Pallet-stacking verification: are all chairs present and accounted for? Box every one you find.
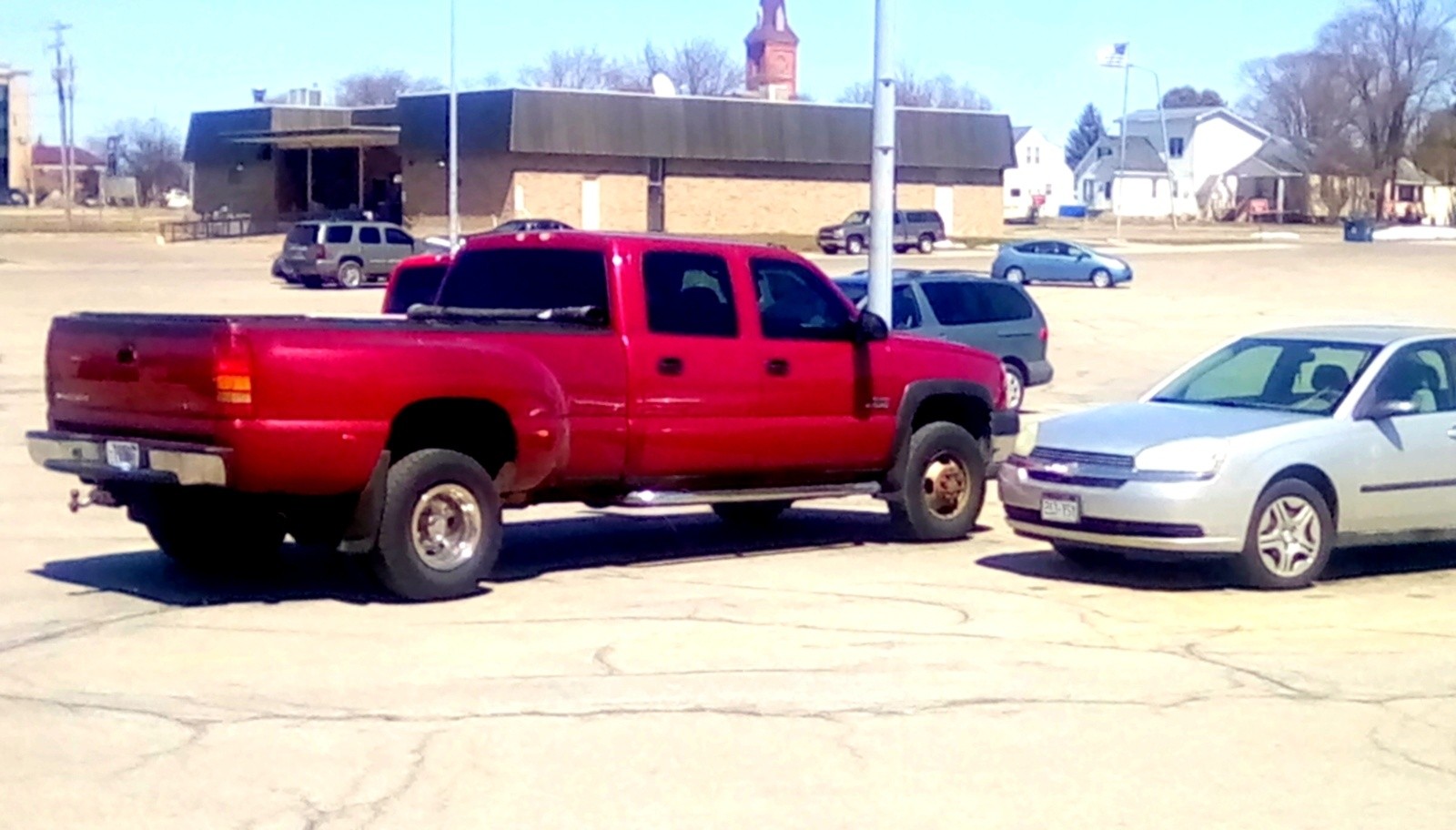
[1311,364,1349,390]
[681,287,719,305]
[1403,362,1440,391]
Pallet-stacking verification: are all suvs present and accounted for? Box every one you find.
[273,219,575,289]
[817,210,947,254]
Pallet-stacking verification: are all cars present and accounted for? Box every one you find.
[991,240,1133,288]
[997,326,1456,589]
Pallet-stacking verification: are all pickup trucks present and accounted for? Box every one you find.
[25,232,1020,603]
